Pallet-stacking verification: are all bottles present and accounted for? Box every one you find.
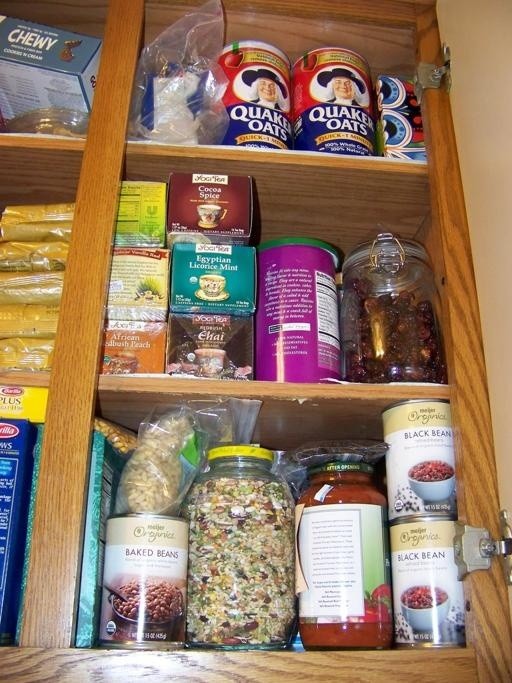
[340,231,447,385]
[188,444,295,651]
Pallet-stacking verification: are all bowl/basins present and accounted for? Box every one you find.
[398,587,449,631]
[408,461,457,502]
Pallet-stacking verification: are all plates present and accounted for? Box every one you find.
[8,106,89,140]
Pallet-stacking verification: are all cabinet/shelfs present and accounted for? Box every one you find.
[0,0,512,683]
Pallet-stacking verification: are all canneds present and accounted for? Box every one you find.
[214,39,290,149]
[98,514,190,651]
[290,47,379,157]
